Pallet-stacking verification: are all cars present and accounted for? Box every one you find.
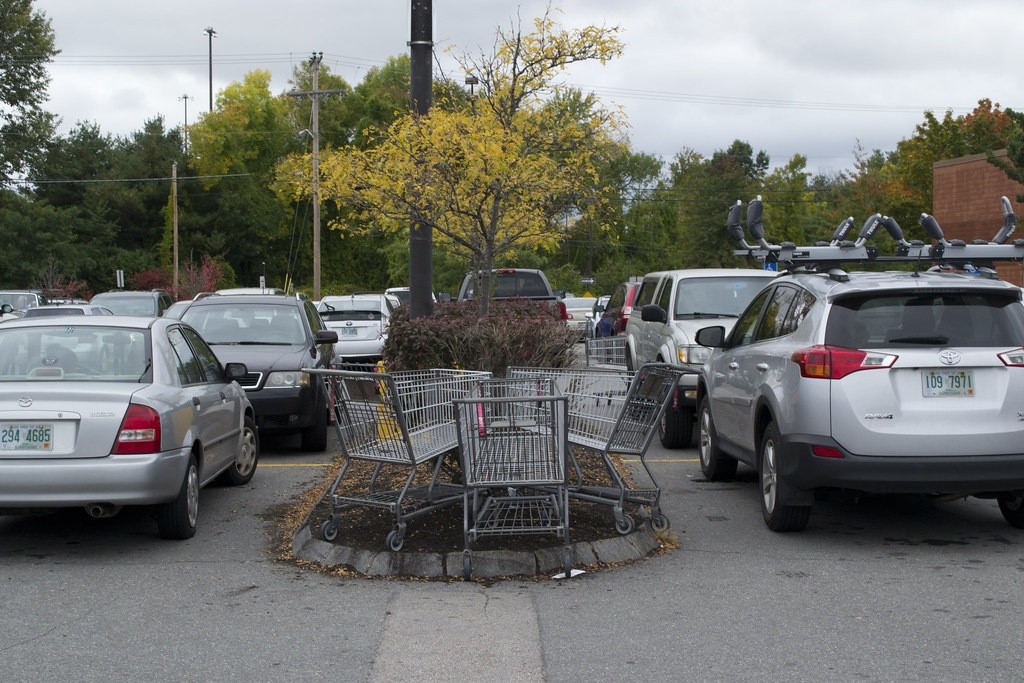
[385,294,403,308]
[0,316,259,540]
[561,298,597,337]
[22,305,115,316]
[162,300,193,319]
[385,286,437,304]
[593,281,644,366]
[317,294,394,370]
[585,295,612,341]
[177,291,337,454]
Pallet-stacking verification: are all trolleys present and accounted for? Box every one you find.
[301,356,491,553]
[450,372,574,581]
[505,365,705,535]
[585,335,625,368]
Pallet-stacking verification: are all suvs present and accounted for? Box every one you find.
[625,268,792,449]
[0,289,48,312]
[697,196,1024,533]
[88,289,173,317]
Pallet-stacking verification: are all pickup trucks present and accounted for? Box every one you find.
[438,267,567,327]
